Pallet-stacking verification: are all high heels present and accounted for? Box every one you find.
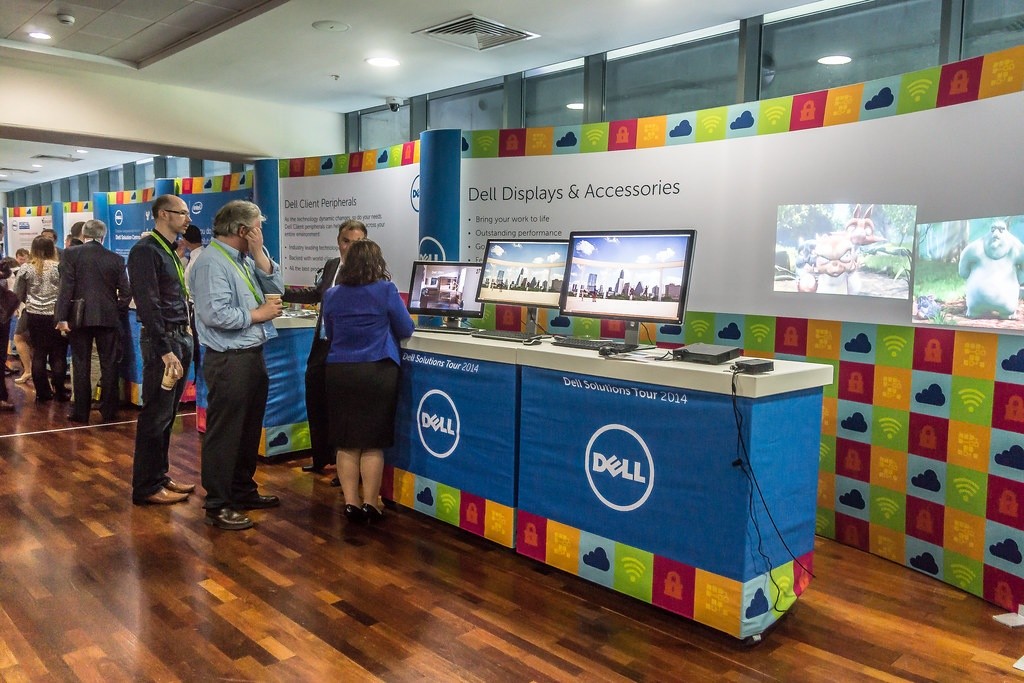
[359,503,388,530]
[344,503,361,531]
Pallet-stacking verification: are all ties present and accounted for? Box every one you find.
[320,266,343,340]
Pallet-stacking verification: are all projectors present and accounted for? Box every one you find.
[672,342,740,364]
[736,359,774,373]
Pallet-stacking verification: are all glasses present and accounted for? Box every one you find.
[165,210,191,217]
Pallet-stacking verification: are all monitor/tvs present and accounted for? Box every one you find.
[475,239,569,334]
[406,261,485,327]
[560,229,697,350]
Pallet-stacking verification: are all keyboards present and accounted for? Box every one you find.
[472,330,542,342]
[550,338,639,353]
[414,326,479,334]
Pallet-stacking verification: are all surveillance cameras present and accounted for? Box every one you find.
[385,97,403,112]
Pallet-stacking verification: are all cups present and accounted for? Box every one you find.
[161,368,179,391]
[264,294,281,302]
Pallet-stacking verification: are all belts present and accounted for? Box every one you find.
[142,320,186,333]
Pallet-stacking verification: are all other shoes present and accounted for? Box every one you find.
[0,400,16,412]
[14,371,32,383]
[100,407,117,423]
[36,385,72,402]
[66,412,89,425]
[4,364,19,376]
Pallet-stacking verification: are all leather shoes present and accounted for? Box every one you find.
[132,486,190,505]
[230,492,279,509]
[302,464,323,472]
[330,476,341,487]
[203,507,254,530]
[164,480,196,493]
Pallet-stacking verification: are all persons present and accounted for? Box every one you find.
[320,240,414,523]
[14,234,70,401]
[41,222,100,402]
[55,220,132,424]
[281,221,368,487]
[127,194,196,504]
[0,222,31,410]
[189,201,285,530]
[177,225,206,384]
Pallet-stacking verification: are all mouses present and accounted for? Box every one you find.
[599,347,612,355]
[523,339,542,345]
